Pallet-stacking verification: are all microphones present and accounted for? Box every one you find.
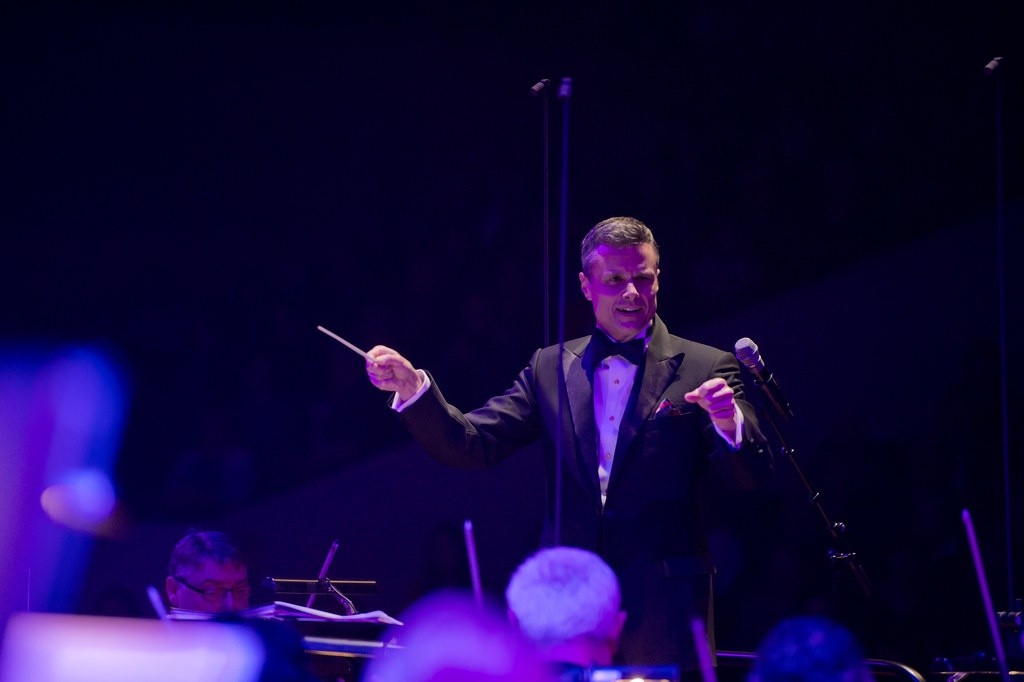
[735,337,793,420]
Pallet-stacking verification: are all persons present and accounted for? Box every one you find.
[366,214,776,680]
[505,545,630,668]
[163,532,249,615]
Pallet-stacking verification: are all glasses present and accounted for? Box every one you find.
[174,576,250,604]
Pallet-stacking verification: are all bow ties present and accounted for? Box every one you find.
[586,330,645,367]
